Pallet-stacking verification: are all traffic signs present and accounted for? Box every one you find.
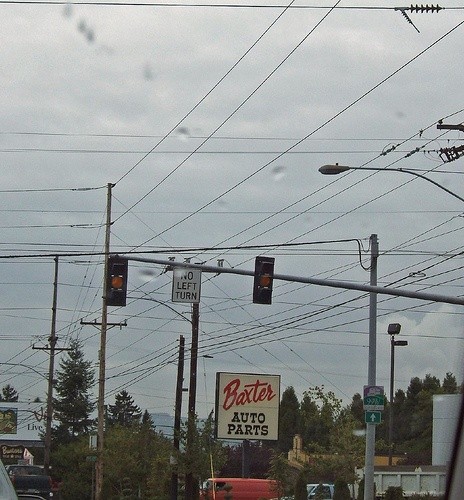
[171,268,201,303]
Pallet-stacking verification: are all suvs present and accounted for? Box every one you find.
[280,482,350,500]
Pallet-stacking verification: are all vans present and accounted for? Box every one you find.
[198,478,283,500]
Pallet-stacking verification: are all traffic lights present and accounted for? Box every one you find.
[252,256,275,305]
[105,257,128,307]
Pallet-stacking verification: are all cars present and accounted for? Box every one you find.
[3,465,52,499]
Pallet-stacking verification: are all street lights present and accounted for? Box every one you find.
[387,323,408,466]
[316,163,464,205]
